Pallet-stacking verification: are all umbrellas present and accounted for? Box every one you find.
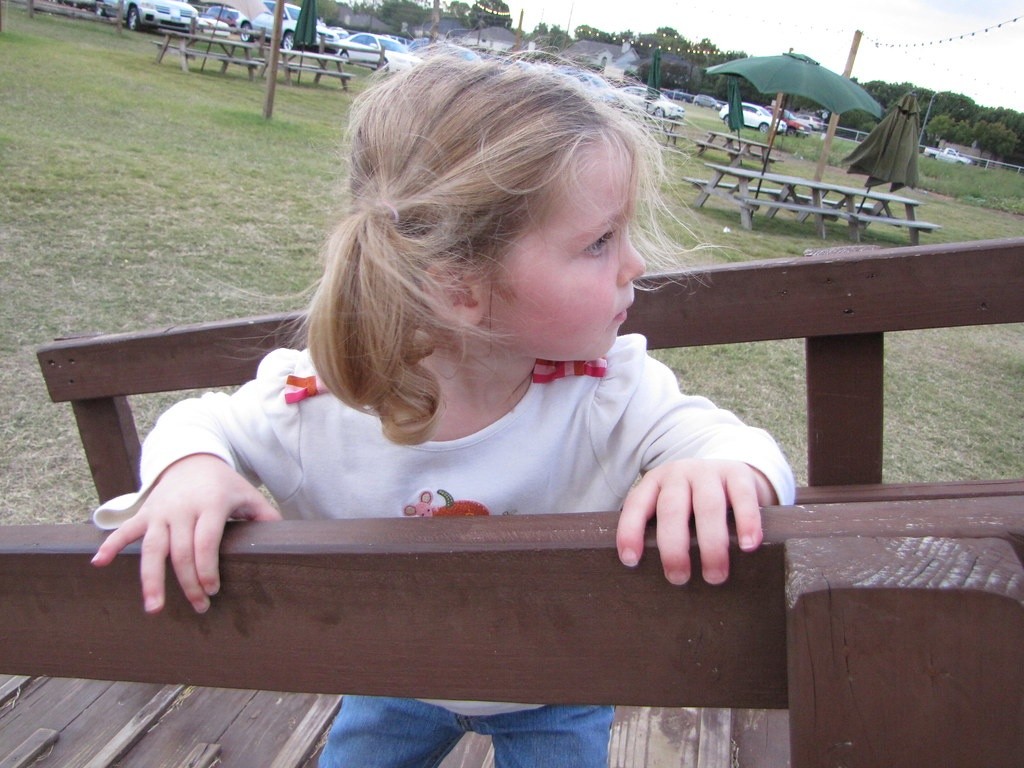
[294,0,317,83]
[643,48,661,118]
[195,0,276,73]
[726,76,745,167]
[838,89,922,214]
[703,53,882,220]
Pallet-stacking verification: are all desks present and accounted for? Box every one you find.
[156,27,255,82]
[693,162,838,240]
[259,45,349,91]
[699,130,774,174]
[797,182,926,245]
[645,109,686,146]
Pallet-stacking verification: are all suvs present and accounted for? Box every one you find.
[235,0,341,55]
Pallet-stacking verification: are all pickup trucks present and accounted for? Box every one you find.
[924,146,971,166]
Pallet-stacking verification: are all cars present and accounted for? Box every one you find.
[693,94,722,111]
[796,114,824,132]
[330,23,479,72]
[504,48,694,121]
[718,102,788,135]
[764,105,811,139]
[197,6,239,38]
[96,0,200,33]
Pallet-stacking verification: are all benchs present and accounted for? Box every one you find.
[152,40,942,232]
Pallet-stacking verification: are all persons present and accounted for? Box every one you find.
[89,37,797,767]
